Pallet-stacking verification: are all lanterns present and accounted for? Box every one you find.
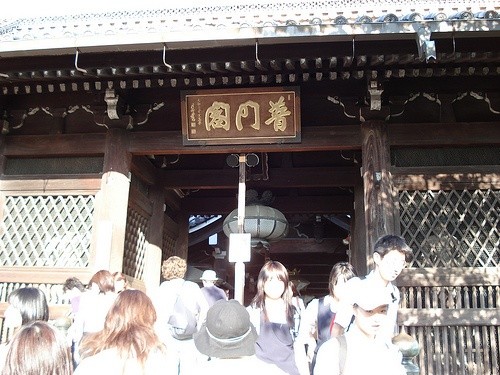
[223,205,288,251]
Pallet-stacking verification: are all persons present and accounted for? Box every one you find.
[313,280,407,375]
[0,256,358,375]
[330,234,413,346]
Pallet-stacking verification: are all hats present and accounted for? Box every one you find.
[199,270,218,281]
[351,280,392,312]
[193,299,257,357]
